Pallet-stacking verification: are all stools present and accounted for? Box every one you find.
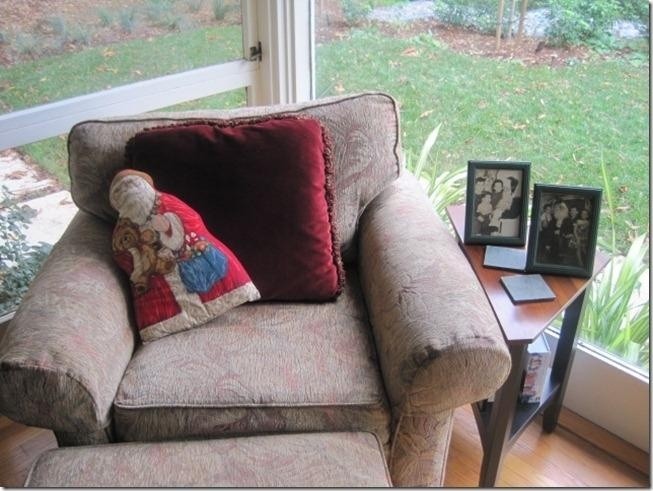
[23,430,393,490]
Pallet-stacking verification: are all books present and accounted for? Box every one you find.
[515,333,551,406]
[499,273,556,305]
[482,246,527,274]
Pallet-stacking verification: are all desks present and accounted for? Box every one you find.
[446,205,611,487]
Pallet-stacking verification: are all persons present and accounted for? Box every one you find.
[472,176,522,237]
[537,201,591,265]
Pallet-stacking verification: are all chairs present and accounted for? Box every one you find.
[1,92,514,486]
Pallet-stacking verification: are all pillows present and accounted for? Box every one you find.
[121,116,344,303]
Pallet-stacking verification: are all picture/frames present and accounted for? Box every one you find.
[465,160,604,279]
